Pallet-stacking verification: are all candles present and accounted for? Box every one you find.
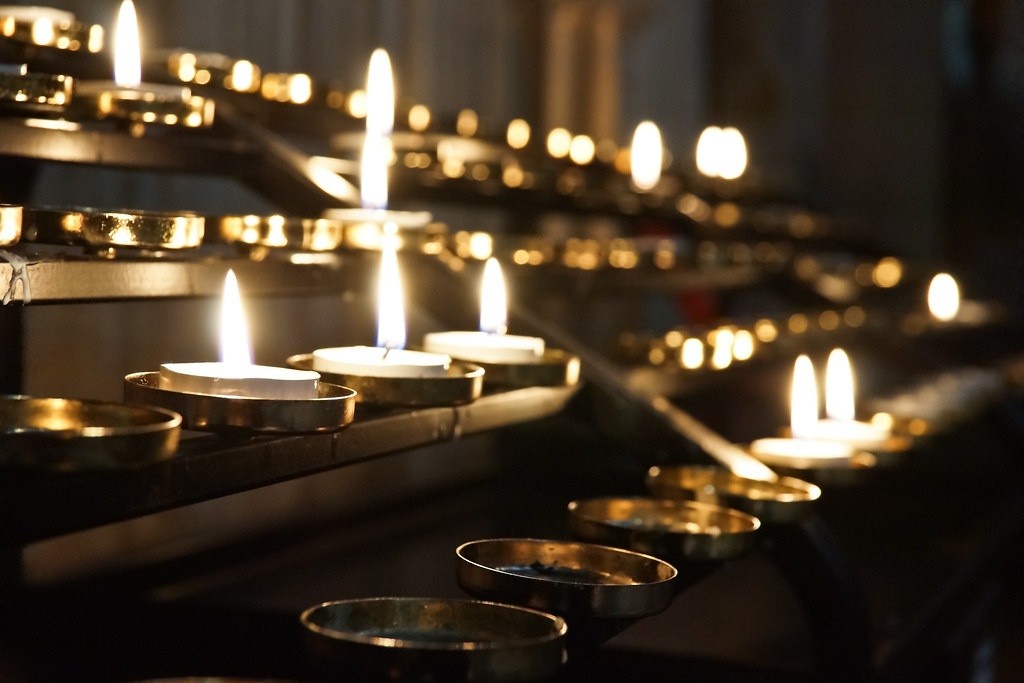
[749,339,882,467]
[325,131,429,250]
[424,257,546,364]
[161,268,322,400]
[76,0,191,103]
[311,250,454,379]
